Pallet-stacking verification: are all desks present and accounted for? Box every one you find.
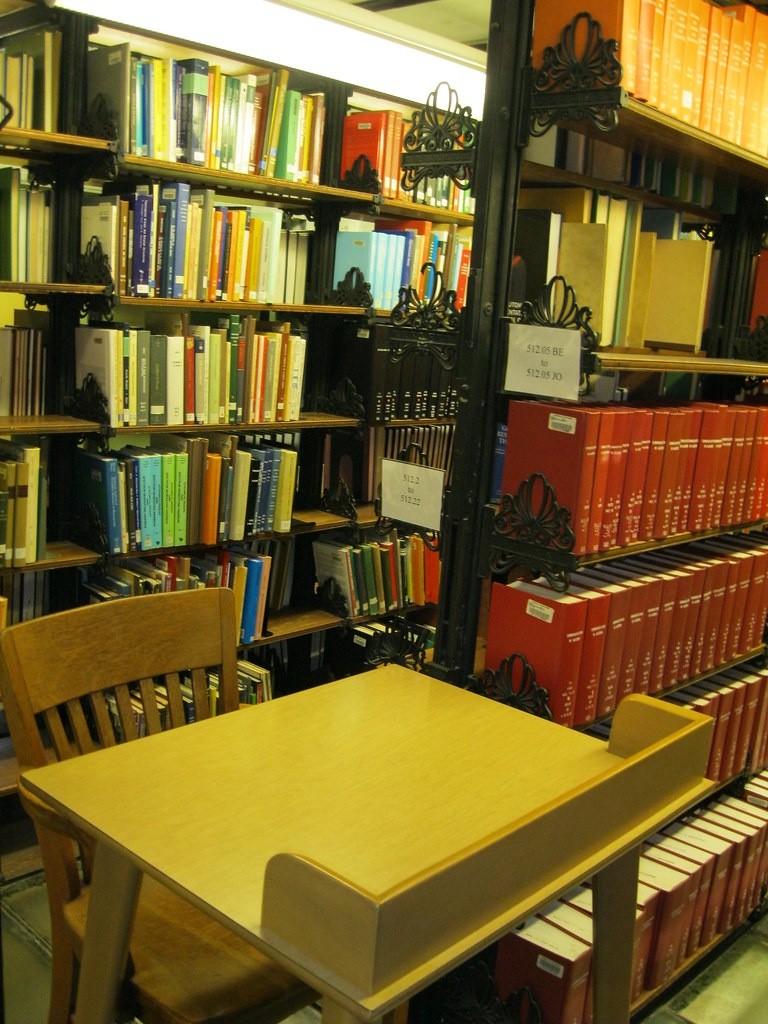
[18,664,719,1024]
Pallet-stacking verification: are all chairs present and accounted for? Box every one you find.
[0,588,413,1024]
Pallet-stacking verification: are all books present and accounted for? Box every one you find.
[1,0,768,1024]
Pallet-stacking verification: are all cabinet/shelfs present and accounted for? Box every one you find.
[0,1,489,887]
[421,1,767,1024]
